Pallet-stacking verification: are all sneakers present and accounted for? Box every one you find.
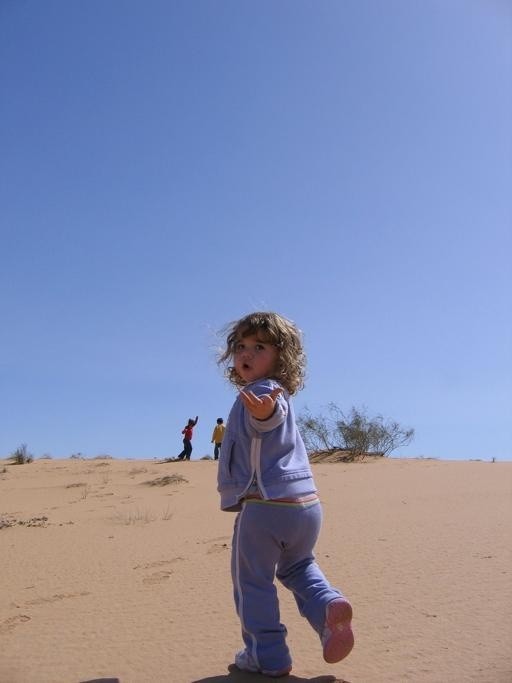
[235,650,292,676]
[320,598,354,663]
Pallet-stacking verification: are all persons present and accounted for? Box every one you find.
[175,415,201,460]
[210,416,226,460]
[213,309,356,677]
